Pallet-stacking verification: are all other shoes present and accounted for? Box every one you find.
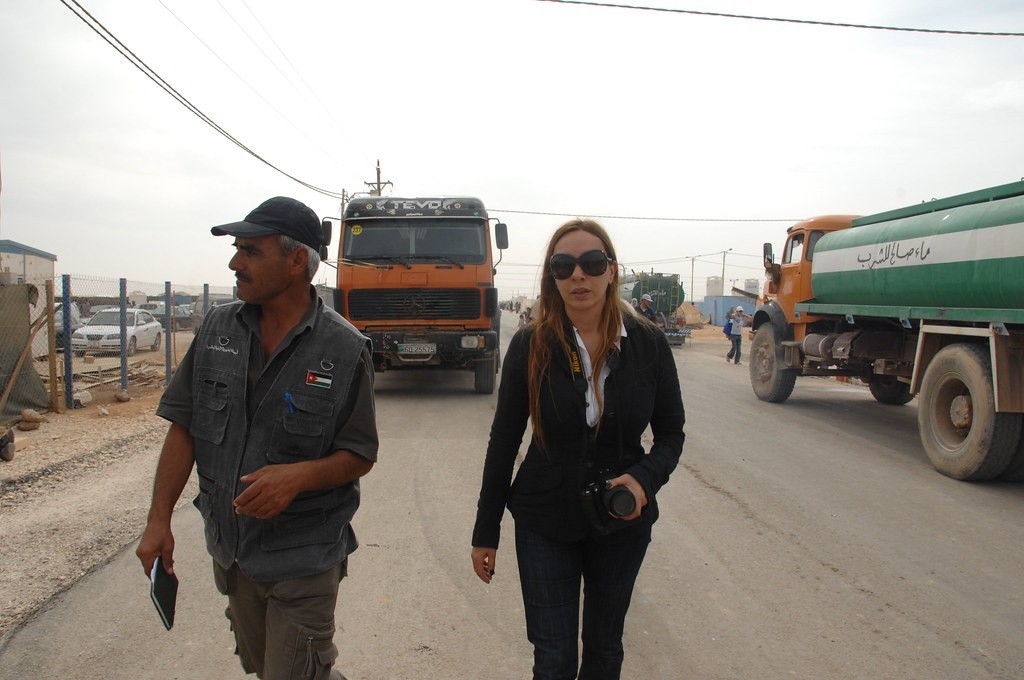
[736,362,742,365]
[726,355,730,362]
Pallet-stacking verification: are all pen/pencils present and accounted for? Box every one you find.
[284,392,294,414]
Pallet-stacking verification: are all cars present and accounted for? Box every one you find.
[53,311,86,348]
[71,307,162,357]
[151,306,193,332]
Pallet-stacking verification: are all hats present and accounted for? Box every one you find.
[211,196,321,252]
[642,294,653,302]
[737,306,743,312]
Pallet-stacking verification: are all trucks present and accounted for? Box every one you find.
[318,196,509,395]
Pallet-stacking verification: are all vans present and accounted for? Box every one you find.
[133,304,157,312]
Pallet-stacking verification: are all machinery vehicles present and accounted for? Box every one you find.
[748,178,1023,483]
[618,272,692,346]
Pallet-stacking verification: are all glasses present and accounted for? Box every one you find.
[549,250,613,280]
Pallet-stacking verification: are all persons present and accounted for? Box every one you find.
[471,218,686,680]
[635,294,656,324]
[501,301,521,314]
[135,197,379,679]
[518,307,532,328]
[726,306,744,365]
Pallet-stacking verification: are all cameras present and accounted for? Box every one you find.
[583,469,636,517]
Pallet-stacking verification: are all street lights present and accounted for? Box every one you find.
[686,254,701,302]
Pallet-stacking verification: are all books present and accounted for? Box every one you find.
[149,558,179,631]
